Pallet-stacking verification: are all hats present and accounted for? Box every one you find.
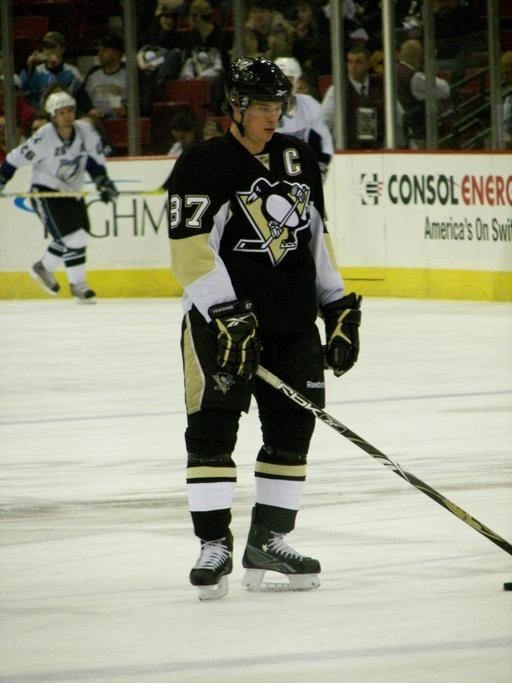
[92,33,124,54]
[40,31,65,45]
[169,112,193,129]
[180,0,212,15]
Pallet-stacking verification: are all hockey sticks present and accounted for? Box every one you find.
[1,173,172,199]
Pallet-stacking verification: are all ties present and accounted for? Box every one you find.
[360,85,366,95]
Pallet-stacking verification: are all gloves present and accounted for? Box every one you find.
[208,299,260,393]
[318,291,362,376]
[97,179,118,203]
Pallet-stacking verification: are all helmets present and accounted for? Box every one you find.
[275,57,301,78]
[155,1,181,17]
[46,92,75,115]
[224,57,293,121]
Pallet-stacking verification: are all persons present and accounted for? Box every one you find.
[275,56,334,191]
[0,0,511,152]
[156,55,363,586]
[2,89,121,304]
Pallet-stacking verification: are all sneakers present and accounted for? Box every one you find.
[70,281,95,299]
[242,528,320,574]
[32,262,60,292]
[189,531,233,586]
